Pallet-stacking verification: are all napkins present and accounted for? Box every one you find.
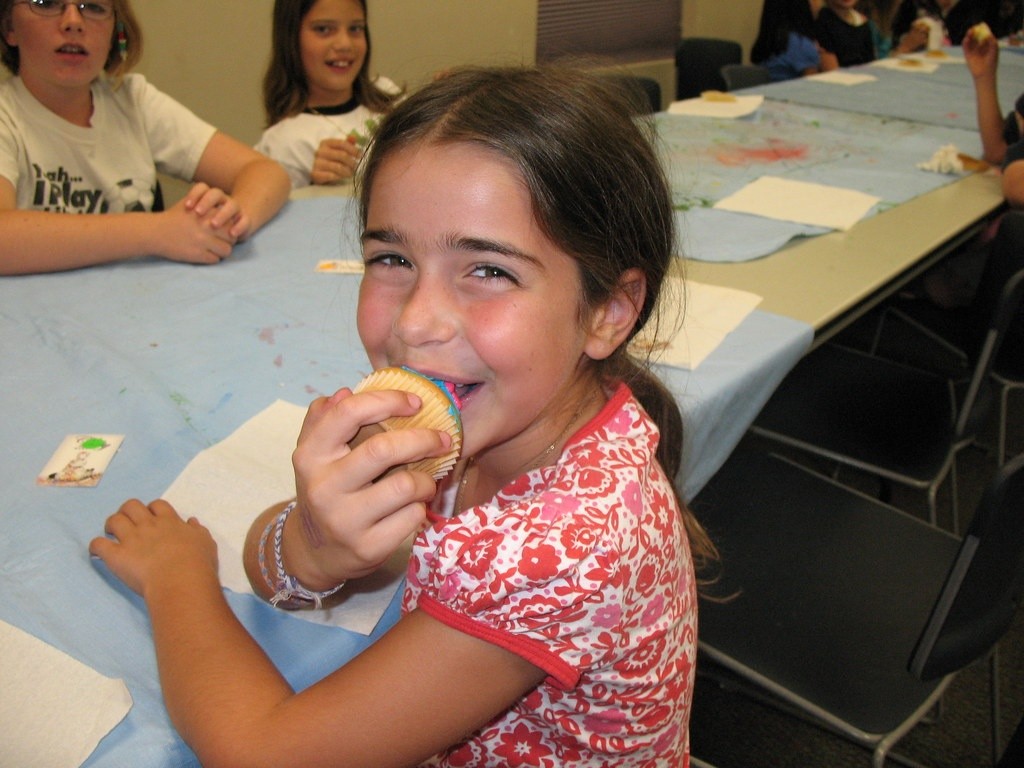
[668,94,764,118]
[618,273,763,372]
[803,68,878,86]
[914,17,943,49]
[712,173,885,233]
[904,52,966,65]
[0,616,136,768]
[160,398,415,636]
[875,57,939,74]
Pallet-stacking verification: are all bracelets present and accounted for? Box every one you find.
[258,502,345,608]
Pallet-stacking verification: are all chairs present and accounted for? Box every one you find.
[684,448,1024,768]
[867,208,1024,470]
[714,215,1024,768]
[750,266,1024,533]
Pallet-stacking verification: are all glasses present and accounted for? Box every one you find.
[13,0,114,20]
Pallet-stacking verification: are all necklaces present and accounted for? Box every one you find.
[310,105,349,137]
[457,383,597,514]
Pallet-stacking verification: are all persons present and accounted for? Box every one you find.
[83,63,721,768]
[749,0,1024,304]
[250,0,413,190]
[0,0,291,276]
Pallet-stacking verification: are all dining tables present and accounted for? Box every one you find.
[0,42,1024,768]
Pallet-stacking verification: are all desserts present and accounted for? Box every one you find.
[345,366,463,481]
